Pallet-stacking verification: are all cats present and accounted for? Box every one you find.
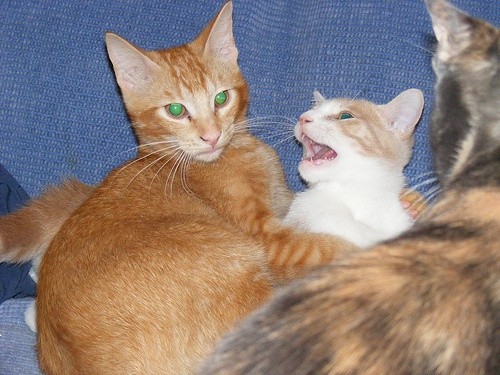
[33,0,360,375]
[0,87,427,338]
[197,1,500,375]
